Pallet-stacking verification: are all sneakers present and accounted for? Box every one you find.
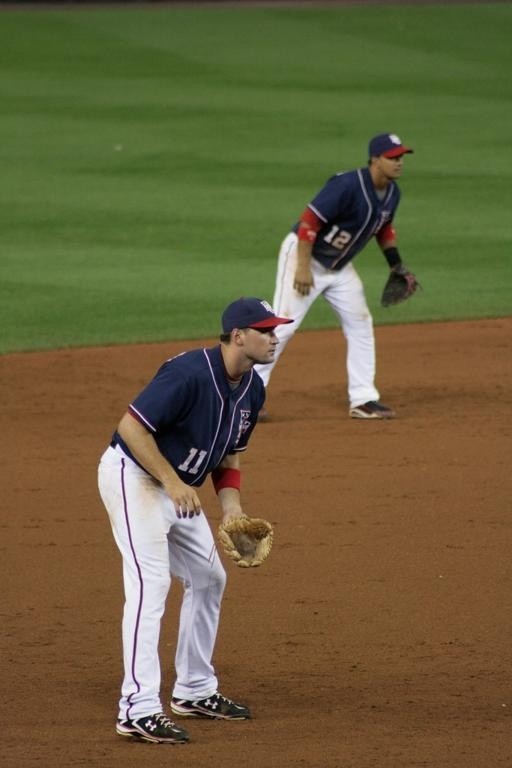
[114,711,189,746]
[349,400,395,420]
[171,691,250,722]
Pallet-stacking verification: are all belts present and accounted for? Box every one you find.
[109,436,117,448]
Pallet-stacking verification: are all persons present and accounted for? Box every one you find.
[96,293,296,744]
[248,130,418,419]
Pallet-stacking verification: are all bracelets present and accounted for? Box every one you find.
[380,244,401,266]
[210,466,244,495]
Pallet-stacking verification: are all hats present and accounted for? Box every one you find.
[222,297,295,333]
[368,132,414,159]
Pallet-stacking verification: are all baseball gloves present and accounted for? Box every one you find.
[382,267,416,307]
[218,514,273,568]
